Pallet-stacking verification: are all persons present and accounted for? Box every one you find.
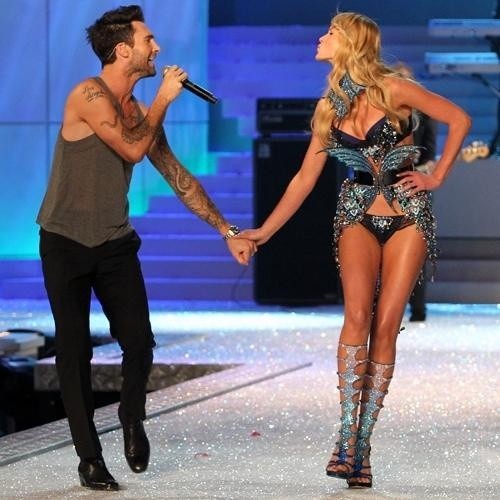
[234,12,473,491]
[38,5,258,491]
[395,58,437,322]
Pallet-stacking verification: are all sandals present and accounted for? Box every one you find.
[325,442,372,489]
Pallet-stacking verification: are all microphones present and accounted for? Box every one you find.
[163,66,218,105]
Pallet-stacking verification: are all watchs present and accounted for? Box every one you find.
[222,225,240,242]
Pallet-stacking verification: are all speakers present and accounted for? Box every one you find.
[253,133,345,305]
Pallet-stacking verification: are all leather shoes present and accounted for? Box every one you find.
[78,458,118,491]
[122,421,150,474]
